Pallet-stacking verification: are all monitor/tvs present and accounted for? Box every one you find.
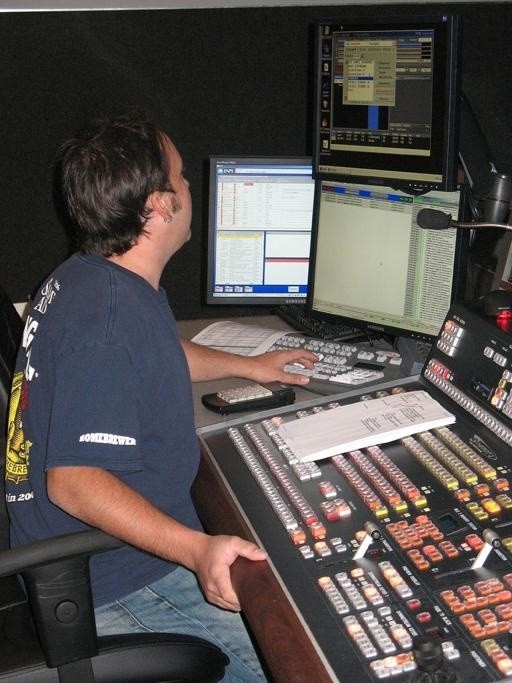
[312,14,464,193]
[305,189,466,345]
[207,155,315,305]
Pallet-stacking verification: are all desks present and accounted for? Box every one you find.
[176,315,512,683]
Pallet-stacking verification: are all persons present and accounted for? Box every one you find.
[4,114,319,682]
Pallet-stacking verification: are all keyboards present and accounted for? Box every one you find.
[267,333,403,397]
[275,305,378,341]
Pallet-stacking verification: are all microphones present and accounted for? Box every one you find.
[417,209,512,230]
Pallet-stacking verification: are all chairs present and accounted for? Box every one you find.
[0,284,231,683]
